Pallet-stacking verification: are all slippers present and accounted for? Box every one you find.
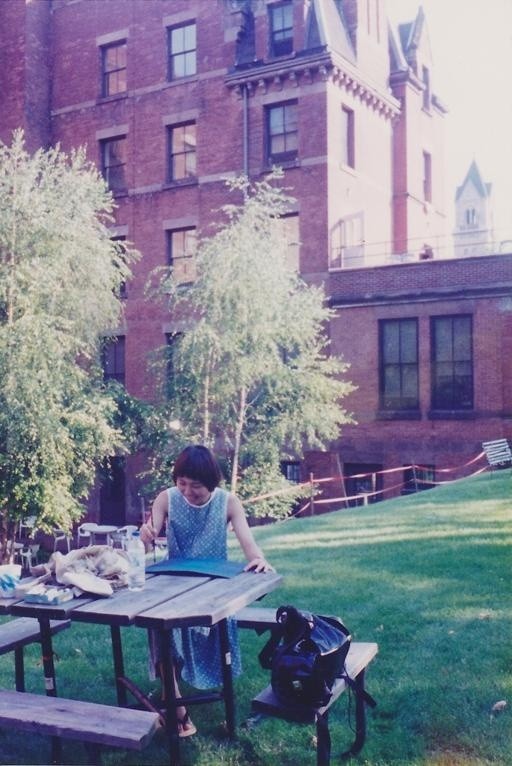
[160,703,198,740]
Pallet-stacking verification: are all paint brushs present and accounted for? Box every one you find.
[150,507,157,566]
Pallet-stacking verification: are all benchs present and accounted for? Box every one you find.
[235,607,287,629]
[0,690,161,766]
[0,616,71,692]
[250,641,379,766]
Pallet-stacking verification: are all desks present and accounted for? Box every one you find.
[0,560,284,766]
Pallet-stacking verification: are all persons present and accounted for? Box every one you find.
[141,445,277,738]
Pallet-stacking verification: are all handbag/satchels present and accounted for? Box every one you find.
[256,602,377,723]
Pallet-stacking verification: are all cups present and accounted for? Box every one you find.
[0,564,22,598]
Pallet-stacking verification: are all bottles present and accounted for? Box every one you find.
[127,532,147,591]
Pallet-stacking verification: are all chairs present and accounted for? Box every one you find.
[6,517,139,571]
